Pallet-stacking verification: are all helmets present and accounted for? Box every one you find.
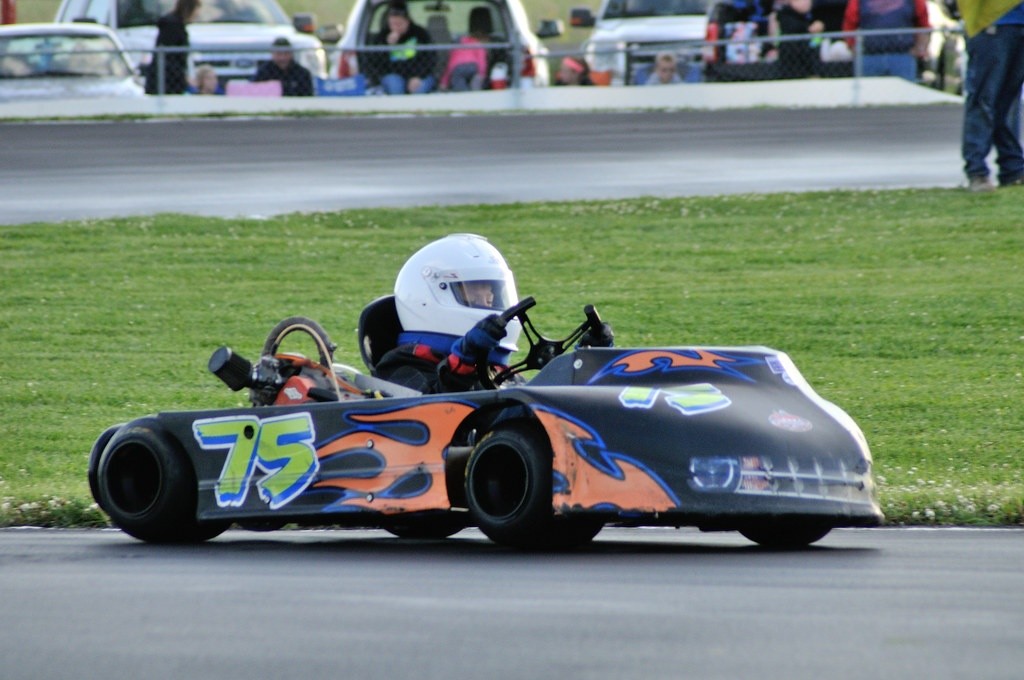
[394,234,522,365]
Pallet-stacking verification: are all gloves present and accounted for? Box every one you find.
[574,322,614,352]
[451,314,507,361]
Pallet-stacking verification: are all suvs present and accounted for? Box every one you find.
[53,0,344,82]
[568,0,721,87]
[318,0,565,95]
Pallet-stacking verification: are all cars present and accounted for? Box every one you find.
[0,22,147,101]
[704,0,969,94]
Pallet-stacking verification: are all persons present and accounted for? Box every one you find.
[197,65,217,94]
[252,39,314,97]
[145,0,202,94]
[703,0,931,84]
[932,0,1024,190]
[366,233,614,399]
[368,6,439,94]
[647,50,682,85]
[440,33,490,91]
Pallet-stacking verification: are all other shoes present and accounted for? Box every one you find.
[971,178,994,191]
[1007,177,1024,186]
